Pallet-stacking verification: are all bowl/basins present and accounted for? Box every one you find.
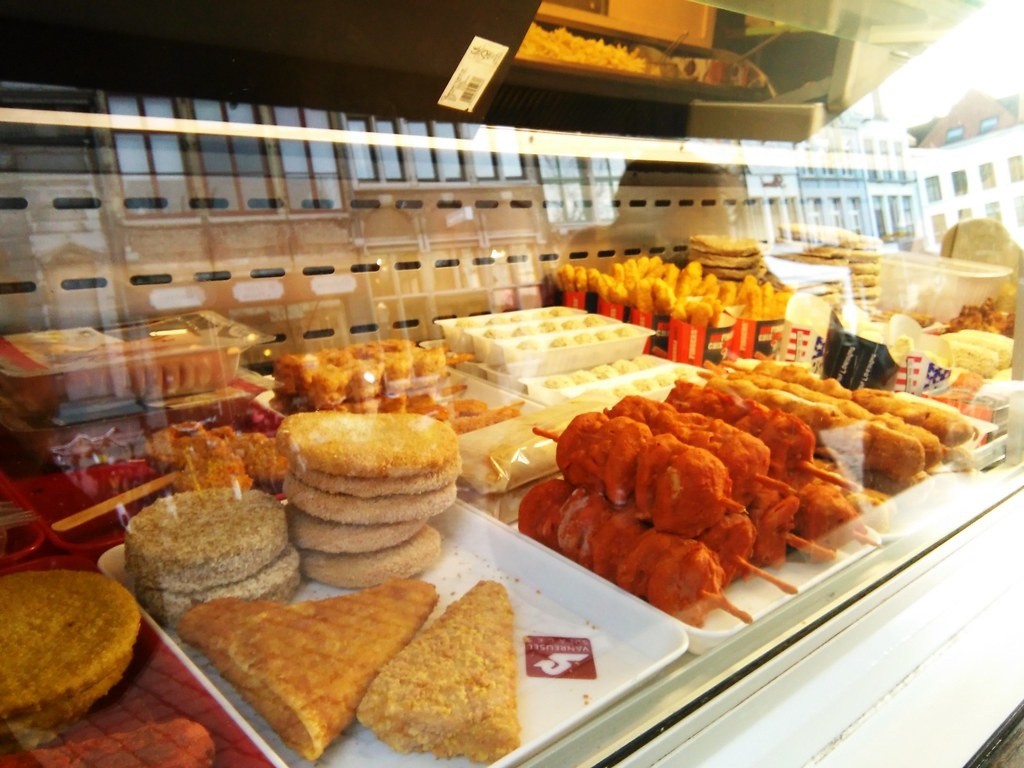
[878,252,1013,324]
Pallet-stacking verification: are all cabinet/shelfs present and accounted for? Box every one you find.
[4,107,1024,768]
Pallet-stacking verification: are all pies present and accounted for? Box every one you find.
[0,411,518,768]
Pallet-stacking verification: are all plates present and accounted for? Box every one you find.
[0,482,45,564]
[99,498,688,768]
[510,519,882,655]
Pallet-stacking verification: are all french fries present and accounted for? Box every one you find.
[552,256,786,332]
